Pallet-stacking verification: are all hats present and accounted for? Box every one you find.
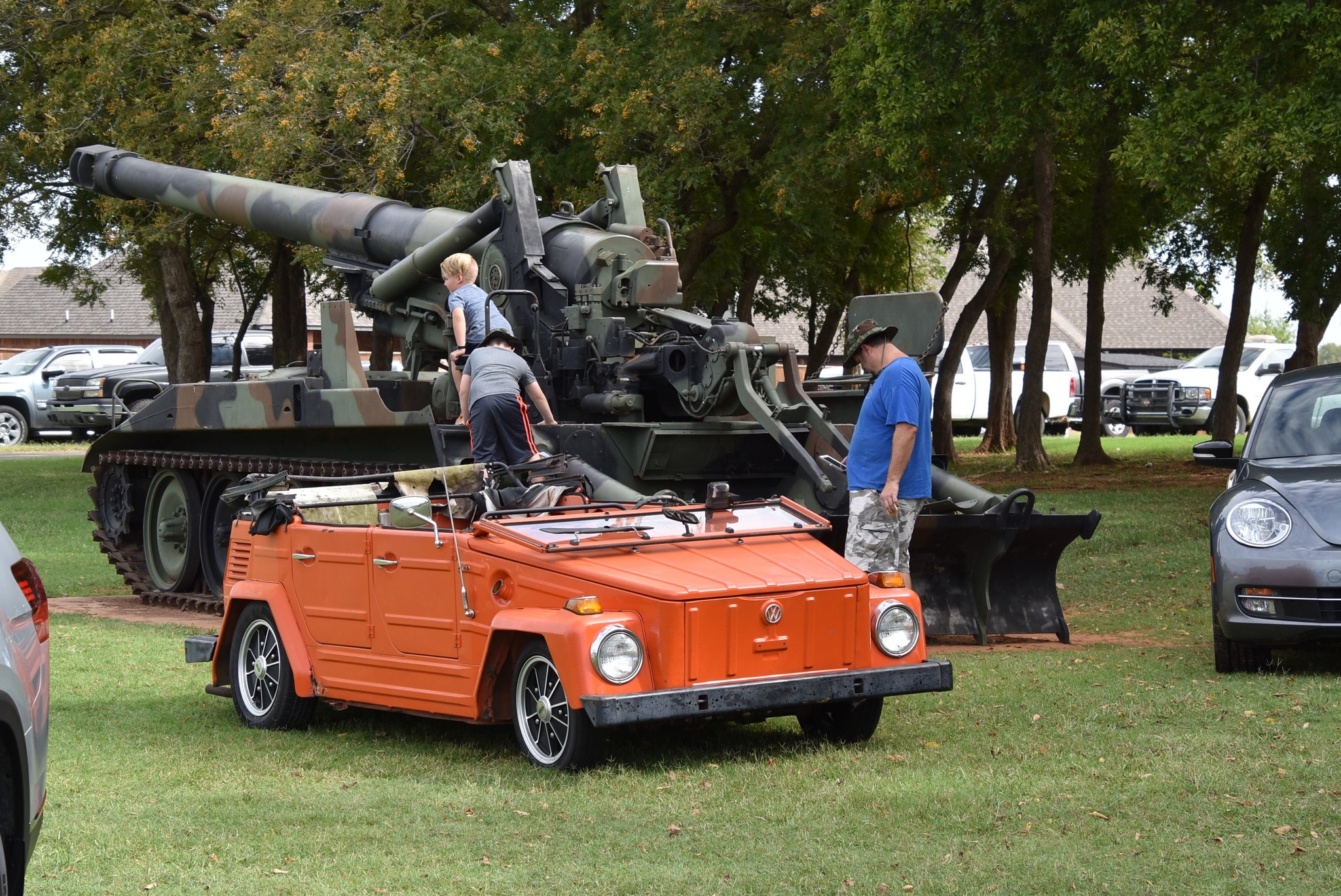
[482,328,524,356]
[842,318,898,371]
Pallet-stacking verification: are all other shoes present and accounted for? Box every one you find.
[455,413,466,426]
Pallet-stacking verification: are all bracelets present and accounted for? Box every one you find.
[456,345,466,349]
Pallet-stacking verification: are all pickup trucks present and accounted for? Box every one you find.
[820,342,1150,437]
[1122,336,1341,436]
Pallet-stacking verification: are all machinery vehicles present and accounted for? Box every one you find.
[62,136,1101,645]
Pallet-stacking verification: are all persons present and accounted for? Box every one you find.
[440,253,515,425]
[833,319,933,593]
[459,332,559,488]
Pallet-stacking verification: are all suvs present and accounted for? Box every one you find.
[0,330,273,445]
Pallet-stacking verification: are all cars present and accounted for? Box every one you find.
[183,457,955,774]
[0,523,50,895]
[1191,363,1341,672]
